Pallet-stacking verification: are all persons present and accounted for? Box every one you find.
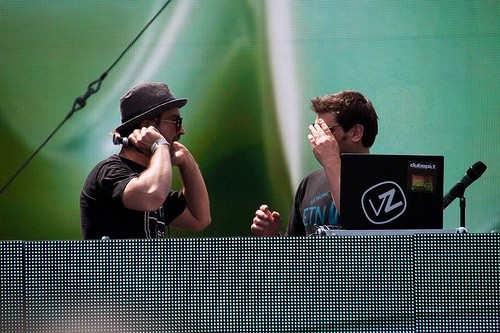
[250,91,379,236]
[80,82,211,239]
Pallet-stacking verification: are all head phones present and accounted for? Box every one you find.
[112,133,144,154]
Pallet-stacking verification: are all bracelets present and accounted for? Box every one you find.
[150,139,171,152]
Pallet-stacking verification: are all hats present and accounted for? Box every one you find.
[114,82,187,132]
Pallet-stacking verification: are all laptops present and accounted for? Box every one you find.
[340,152,444,230]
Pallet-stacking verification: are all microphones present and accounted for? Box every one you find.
[443,161,488,208]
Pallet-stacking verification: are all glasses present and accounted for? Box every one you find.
[312,123,355,134]
[150,117,182,132]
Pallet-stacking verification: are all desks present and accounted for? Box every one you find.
[0,232,500,333]
